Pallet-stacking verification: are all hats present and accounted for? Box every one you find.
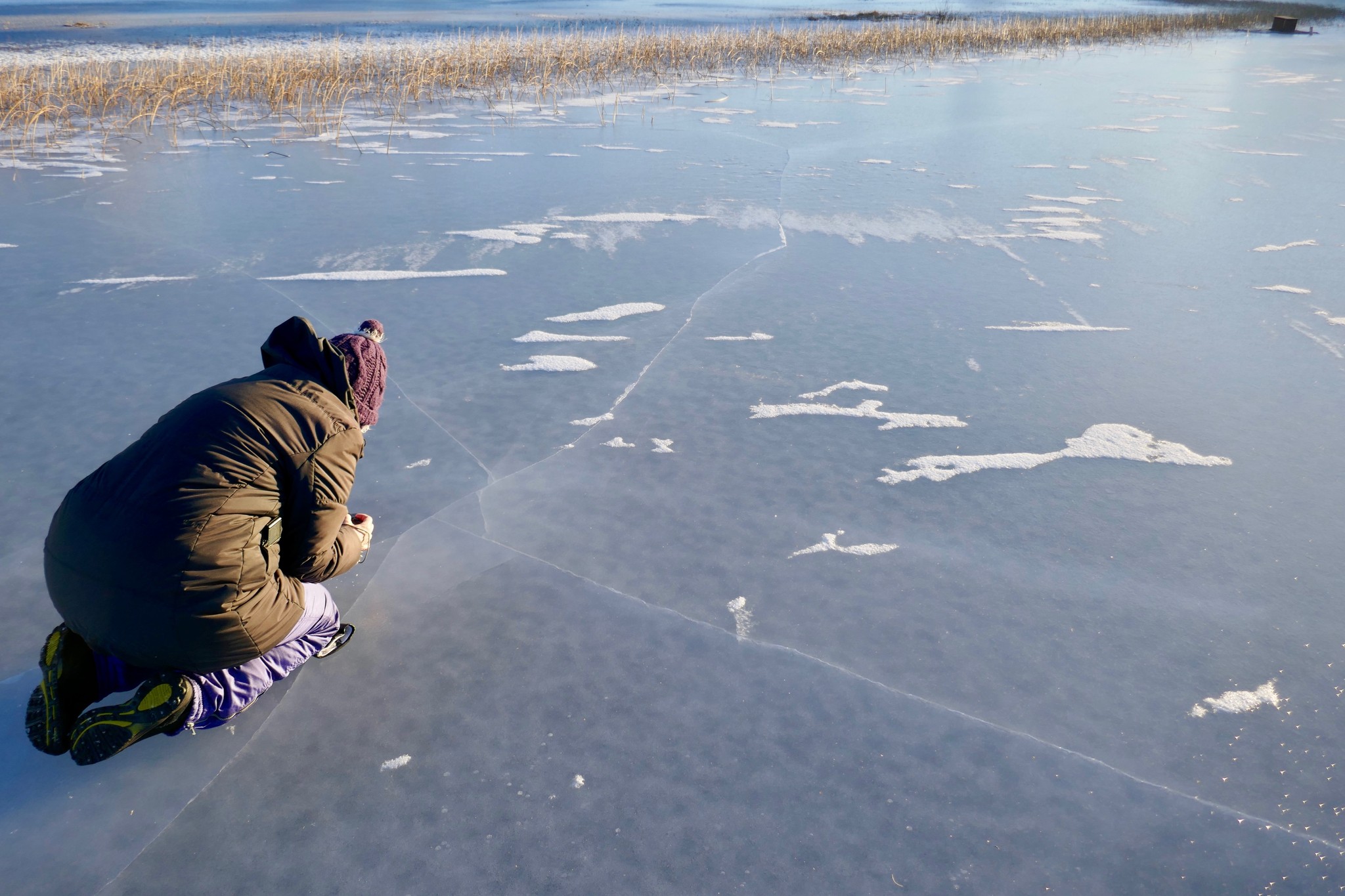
[328,319,386,425]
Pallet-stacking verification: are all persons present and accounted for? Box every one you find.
[24,317,389,768]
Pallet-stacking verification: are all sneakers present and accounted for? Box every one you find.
[25,618,99,756]
[70,672,194,765]
